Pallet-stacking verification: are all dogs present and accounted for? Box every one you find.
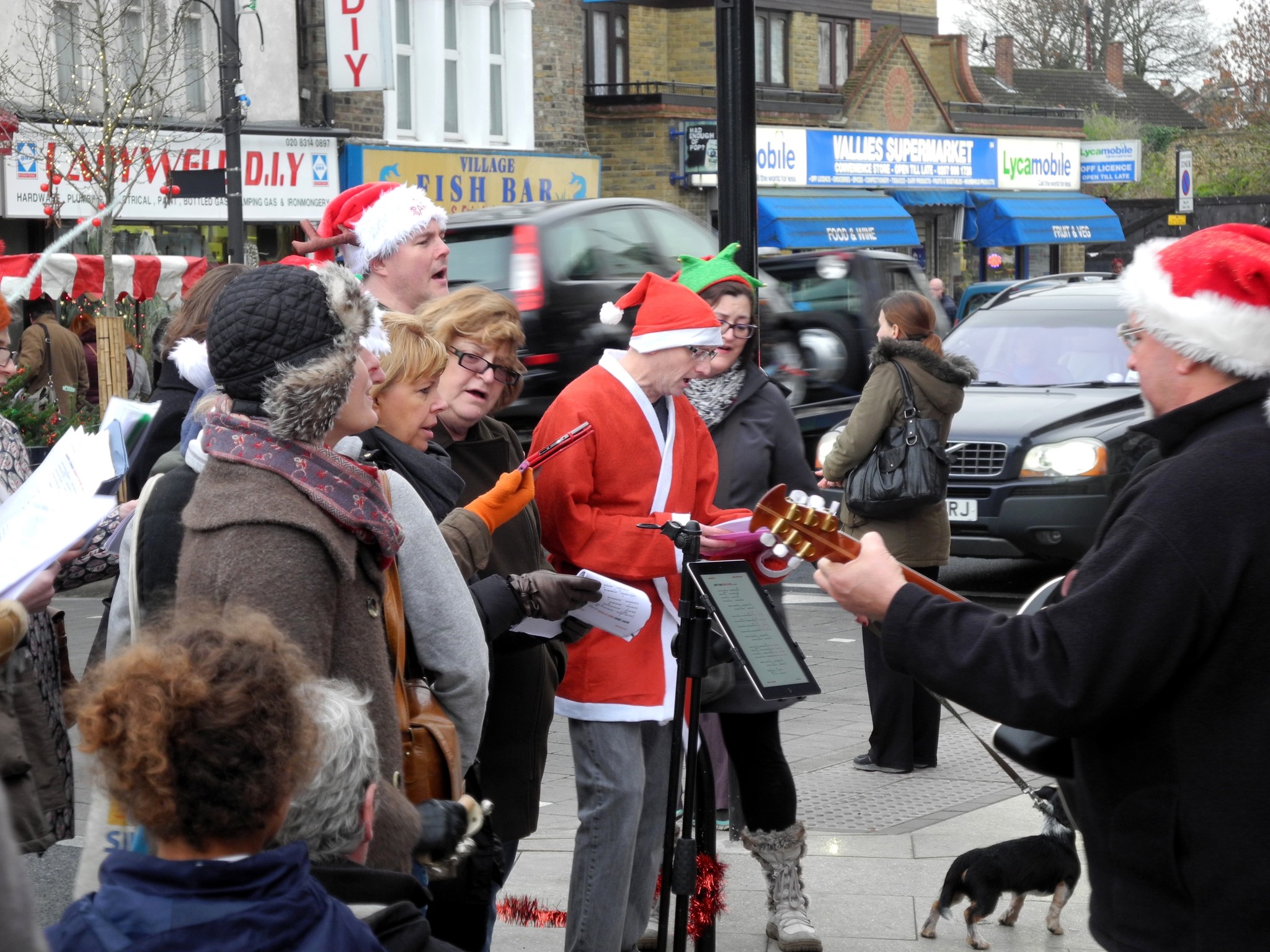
[920,786,1081,950]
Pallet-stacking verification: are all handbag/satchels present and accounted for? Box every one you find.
[845,359,958,518]
[15,324,61,422]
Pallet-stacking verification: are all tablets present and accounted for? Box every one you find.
[526,420,594,470]
[686,560,822,703]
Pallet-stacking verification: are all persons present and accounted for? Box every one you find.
[529,276,770,952]
[815,290,978,773]
[7,181,604,952]
[664,241,825,952]
[814,230,1270,952]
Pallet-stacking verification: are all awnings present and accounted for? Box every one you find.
[885,190,979,243]
[967,191,1125,250]
[756,193,922,252]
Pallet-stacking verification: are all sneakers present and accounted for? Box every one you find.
[853,754,913,773]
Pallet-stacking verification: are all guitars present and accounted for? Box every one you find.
[746,482,1079,781]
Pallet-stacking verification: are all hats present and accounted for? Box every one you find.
[277,255,393,356]
[25,299,57,311]
[204,261,378,443]
[668,242,767,314]
[599,272,724,353]
[1113,222,1270,379]
[292,181,448,280]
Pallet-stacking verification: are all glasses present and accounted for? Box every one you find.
[447,345,520,386]
[711,319,758,340]
[685,343,718,362]
[1116,322,1147,351]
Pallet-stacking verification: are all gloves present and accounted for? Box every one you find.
[507,569,602,622]
[462,467,535,535]
[560,615,594,644]
[417,799,468,862]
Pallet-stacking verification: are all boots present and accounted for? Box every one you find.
[740,823,822,952]
[636,824,679,947]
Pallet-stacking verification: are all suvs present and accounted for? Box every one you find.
[437,194,811,431]
[816,272,1156,569]
[757,243,953,399]
